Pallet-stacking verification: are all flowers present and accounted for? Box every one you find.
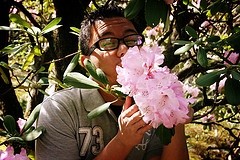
[0,0,240,160]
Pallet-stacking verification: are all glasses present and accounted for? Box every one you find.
[86,33,145,56]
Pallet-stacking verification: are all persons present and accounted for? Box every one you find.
[34,7,194,160]
